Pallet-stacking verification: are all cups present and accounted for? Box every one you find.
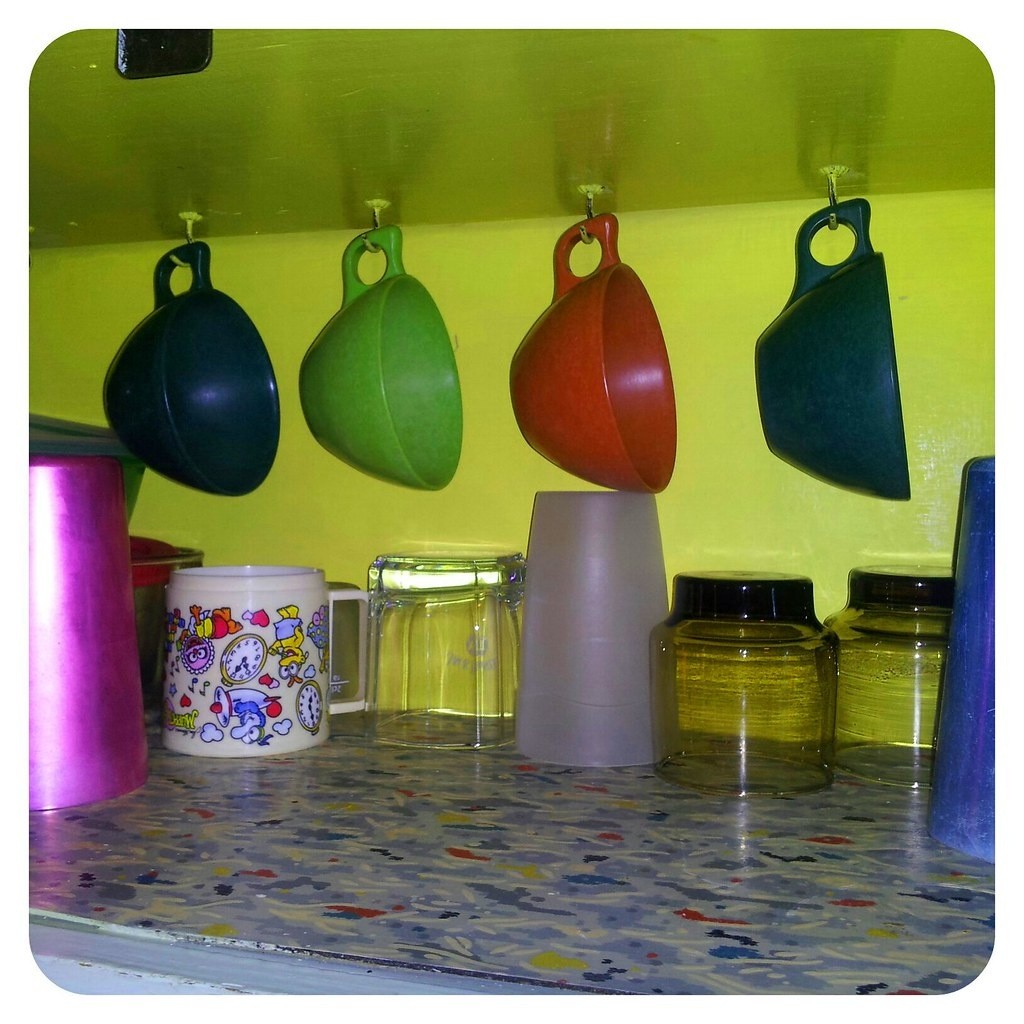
[29,454,154,813]
[926,452,994,865]
[98,241,280,497]
[648,568,839,797]
[507,213,676,495]
[299,225,463,487]
[822,563,955,791]
[754,198,913,503]
[523,486,679,770]
[368,549,527,749]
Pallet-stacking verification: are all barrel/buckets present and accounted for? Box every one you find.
[163,563,377,758]
